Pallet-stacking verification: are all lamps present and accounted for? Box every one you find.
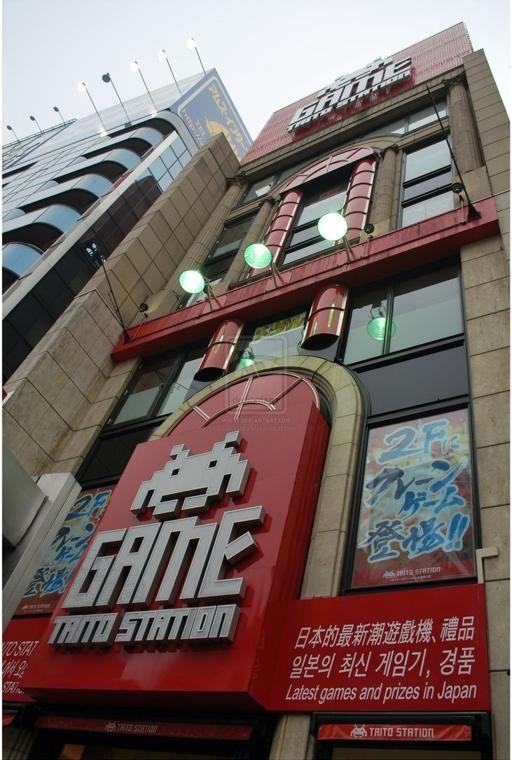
[82,236,133,344]
[102,72,129,116]
[178,211,354,312]
[365,299,398,343]
